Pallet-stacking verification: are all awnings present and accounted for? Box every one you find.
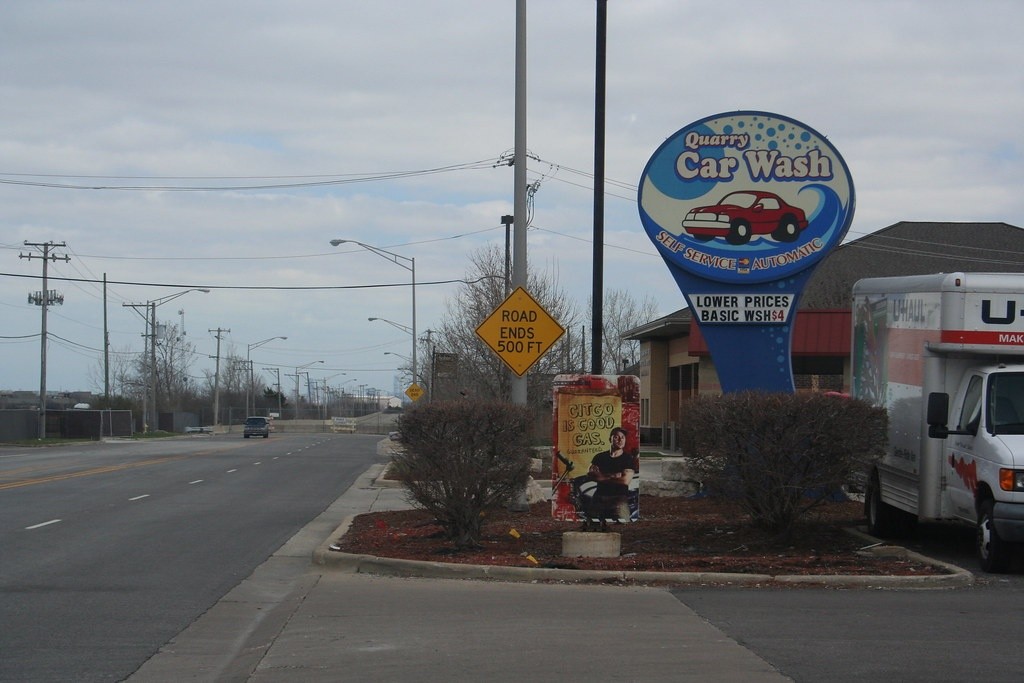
[687,310,851,357]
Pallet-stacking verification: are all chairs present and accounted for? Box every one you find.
[995,397,1017,425]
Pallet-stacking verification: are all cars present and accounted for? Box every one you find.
[243,416,270,438]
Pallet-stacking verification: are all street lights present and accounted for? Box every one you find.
[367,317,432,406]
[247,337,288,418]
[296,360,325,419]
[338,378,357,417]
[142,289,210,435]
[330,239,417,408]
[325,372,346,420]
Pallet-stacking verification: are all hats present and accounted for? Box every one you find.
[610,427,627,436]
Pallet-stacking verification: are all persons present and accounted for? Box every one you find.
[589,428,635,520]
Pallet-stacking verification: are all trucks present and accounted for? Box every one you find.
[848,271,1024,574]
